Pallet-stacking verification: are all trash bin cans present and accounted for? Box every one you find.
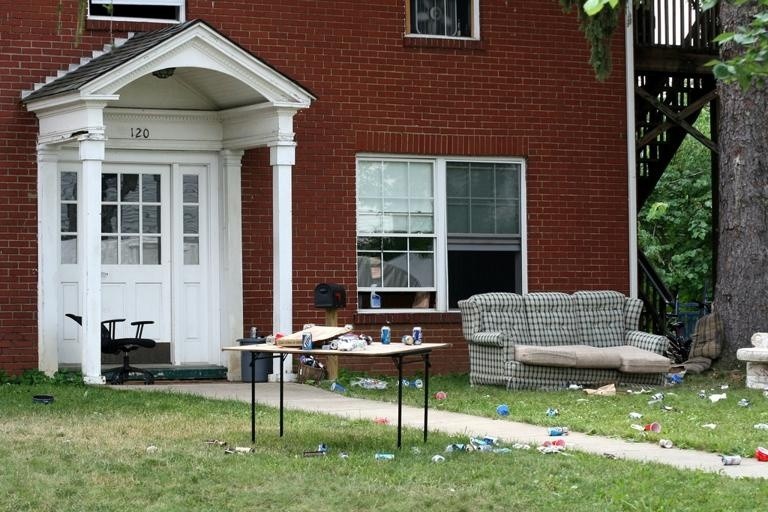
[236,337,268,383]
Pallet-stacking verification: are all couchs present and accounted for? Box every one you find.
[456,289,671,392]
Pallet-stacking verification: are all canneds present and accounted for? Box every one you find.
[629,411,643,419]
[412,326,423,345]
[206,439,254,455]
[721,455,741,465]
[660,439,673,449]
[738,398,752,409]
[375,453,395,461]
[300,354,327,372]
[381,326,391,344]
[431,454,445,463]
[545,408,560,417]
[301,323,372,351]
[762,391,768,398]
[402,335,414,345]
[397,379,410,387]
[304,451,325,457]
[446,436,511,453]
[249,327,258,338]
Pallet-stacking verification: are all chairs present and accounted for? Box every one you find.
[65,313,157,386]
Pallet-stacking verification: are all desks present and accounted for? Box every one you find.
[220,340,455,450]
